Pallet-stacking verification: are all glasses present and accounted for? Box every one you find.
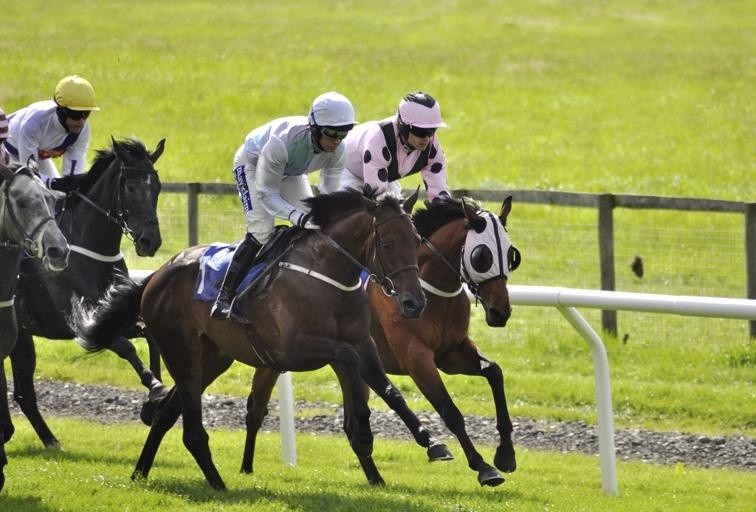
[67,110,90,120]
[410,127,432,137]
[320,127,347,138]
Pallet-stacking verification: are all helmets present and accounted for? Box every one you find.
[55,74,100,111]
[397,92,446,128]
[308,92,359,127]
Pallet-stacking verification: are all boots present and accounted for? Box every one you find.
[210,233,262,324]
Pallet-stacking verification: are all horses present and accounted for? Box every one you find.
[65,184,455,490]
[9,134,167,455]
[239,196,517,487]
[1,143,72,488]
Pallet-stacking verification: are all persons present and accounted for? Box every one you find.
[1,110,10,169]
[1,76,100,196]
[210,90,355,320]
[335,91,452,205]
